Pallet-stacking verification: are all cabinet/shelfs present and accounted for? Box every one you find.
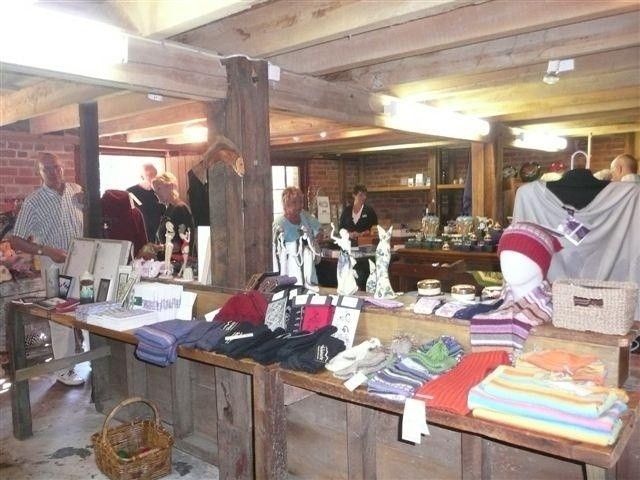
[338,141,473,230]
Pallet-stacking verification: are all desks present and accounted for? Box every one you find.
[7,297,639,479]
[389,247,502,292]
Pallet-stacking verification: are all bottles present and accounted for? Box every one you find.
[44,263,60,300]
[78,271,95,302]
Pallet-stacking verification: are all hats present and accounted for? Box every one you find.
[497,221,563,281]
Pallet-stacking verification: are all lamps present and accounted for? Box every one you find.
[507,127,569,152]
[0,5,130,67]
[125,125,179,144]
[166,125,208,144]
[542,59,561,85]
[373,95,495,143]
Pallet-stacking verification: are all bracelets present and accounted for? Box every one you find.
[160,244,166,251]
[38,245,43,255]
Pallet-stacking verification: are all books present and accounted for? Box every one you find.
[10,264,185,332]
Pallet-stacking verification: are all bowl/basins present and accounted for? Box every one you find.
[483,285,502,300]
[418,278,442,297]
[451,284,476,302]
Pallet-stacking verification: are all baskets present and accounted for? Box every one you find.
[92,397,174,480]
[553,279,637,337]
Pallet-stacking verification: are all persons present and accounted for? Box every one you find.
[595,154,640,184]
[272,187,323,285]
[296,227,321,286]
[10,153,88,386]
[178,223,191,277]
[330,222,358,295]
[163,220,175,277]
[340,185,378,240]
[125,164,166,243]
[145,173,194,263]
[275,226,289,276]
[497,220,563,307]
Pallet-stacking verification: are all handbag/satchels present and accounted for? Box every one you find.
[247,272,297,294]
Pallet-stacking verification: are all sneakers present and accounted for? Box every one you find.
[56,369,84,385]
